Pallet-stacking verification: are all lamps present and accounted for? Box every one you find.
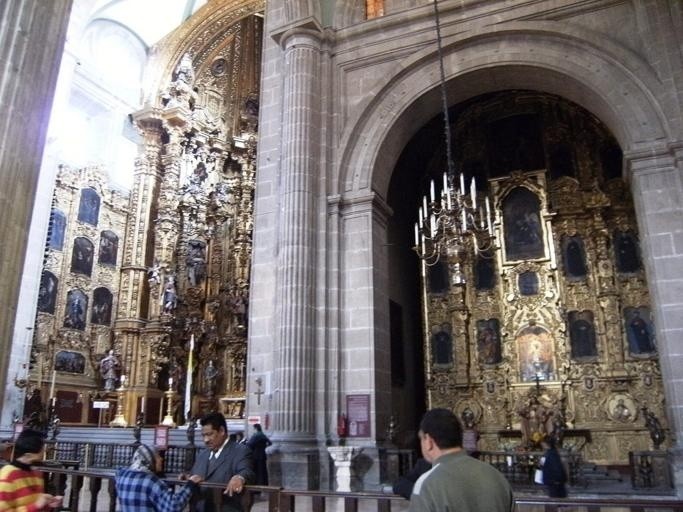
[412,1,503,290]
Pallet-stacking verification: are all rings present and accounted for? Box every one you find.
[190,477,192,479]
[236,487,239,491]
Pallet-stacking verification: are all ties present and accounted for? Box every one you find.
[208,450,217,471]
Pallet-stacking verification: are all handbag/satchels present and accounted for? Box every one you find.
[534,469,544,485]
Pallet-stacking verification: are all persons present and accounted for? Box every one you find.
[0,428,65,512]
[177,411,256,512]
[113,443,202,512]
[541,433,567,498]
[204,359,219,396]
[100,350,119,392]
[161,276,178,316]
[246,422,273,487]
[408,407,518,512]
